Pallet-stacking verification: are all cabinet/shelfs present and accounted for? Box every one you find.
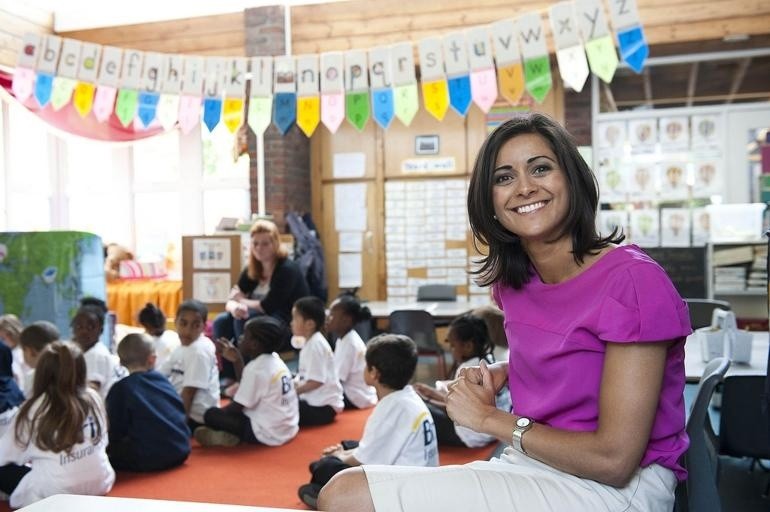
[703,242,768,303]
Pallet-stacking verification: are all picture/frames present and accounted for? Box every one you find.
[414,135,440,155]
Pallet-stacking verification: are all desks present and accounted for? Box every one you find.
[679,331,769,382]
[181,233,295,315]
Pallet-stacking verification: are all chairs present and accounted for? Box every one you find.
[646,336,733,511]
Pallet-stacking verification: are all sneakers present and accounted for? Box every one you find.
[194,427,242,448]
[298,485,325,507]
